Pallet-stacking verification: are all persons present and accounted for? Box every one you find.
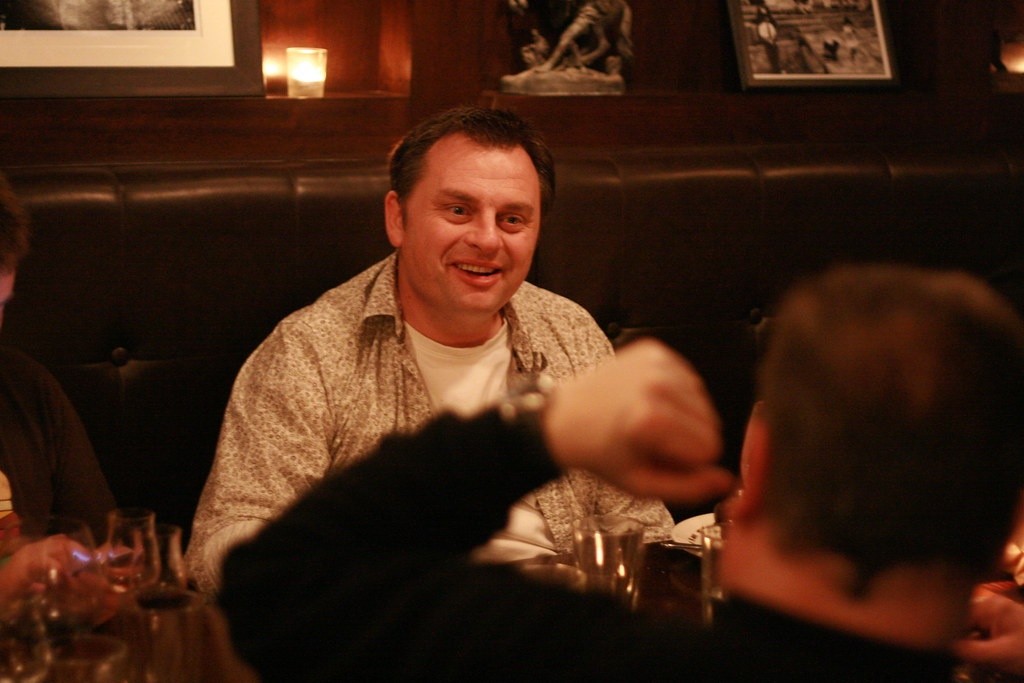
[222,265,1024,683]
[509,0,633,73]
[175,105,670,598]
[0,178,120,624]
[754,0,860,74]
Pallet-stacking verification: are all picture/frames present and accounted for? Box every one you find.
[0,0,270,103]
[725,0,900,89]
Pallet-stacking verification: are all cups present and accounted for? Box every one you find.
[0,592,53,683]
[284,46,329,99]
[102,510,161,594]
[30,519,104,630]
[133,589,203,683]
[571,517,646,611]
[156,526,187,600]
[700,525,723,618]
[33,634,128,683]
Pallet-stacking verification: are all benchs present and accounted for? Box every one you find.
[0,153,1024,584]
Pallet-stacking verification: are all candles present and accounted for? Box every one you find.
[286,47,327,98]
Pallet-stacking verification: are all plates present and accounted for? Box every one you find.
[672,514,716,557]
